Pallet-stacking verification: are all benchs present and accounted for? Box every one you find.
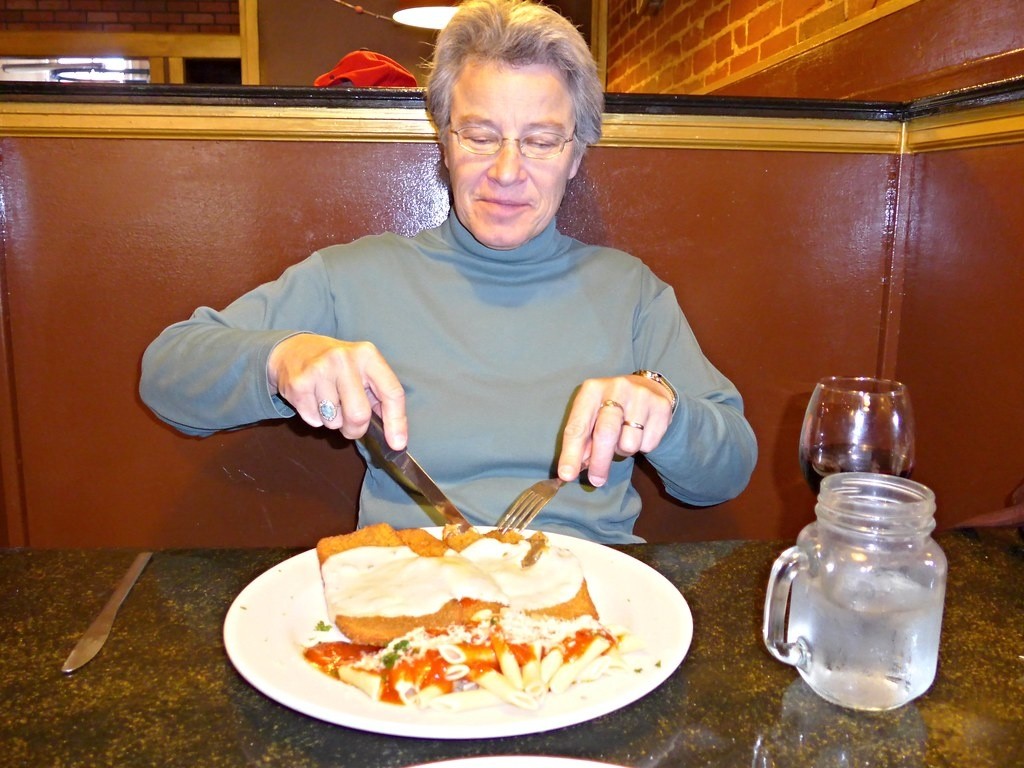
[0,81,902,546]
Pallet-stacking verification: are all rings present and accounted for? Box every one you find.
[623,421,644,430]
[601,400,624,410]
[319,399,341,422]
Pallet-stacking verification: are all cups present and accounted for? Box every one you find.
[799,376,916,496]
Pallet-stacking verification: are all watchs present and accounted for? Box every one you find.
[632,370,675,408]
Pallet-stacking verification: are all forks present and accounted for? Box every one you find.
[494,455,590,534]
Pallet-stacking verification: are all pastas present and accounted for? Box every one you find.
[338,632,646,712]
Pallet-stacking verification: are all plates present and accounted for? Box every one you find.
[223,526,694,739]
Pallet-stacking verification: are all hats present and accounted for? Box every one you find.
[312,48,417,87]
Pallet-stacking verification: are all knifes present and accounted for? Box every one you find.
[62,550,154,672]
[357,409,471,531]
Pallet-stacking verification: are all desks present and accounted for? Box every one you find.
[0,549,1024,768]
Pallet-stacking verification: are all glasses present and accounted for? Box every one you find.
[448,119,576,159]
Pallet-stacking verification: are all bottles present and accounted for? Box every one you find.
[763,471,948,712]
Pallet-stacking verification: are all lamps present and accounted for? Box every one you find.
[393,0,462,31]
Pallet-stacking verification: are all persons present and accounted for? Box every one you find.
[138,0,758,543]
